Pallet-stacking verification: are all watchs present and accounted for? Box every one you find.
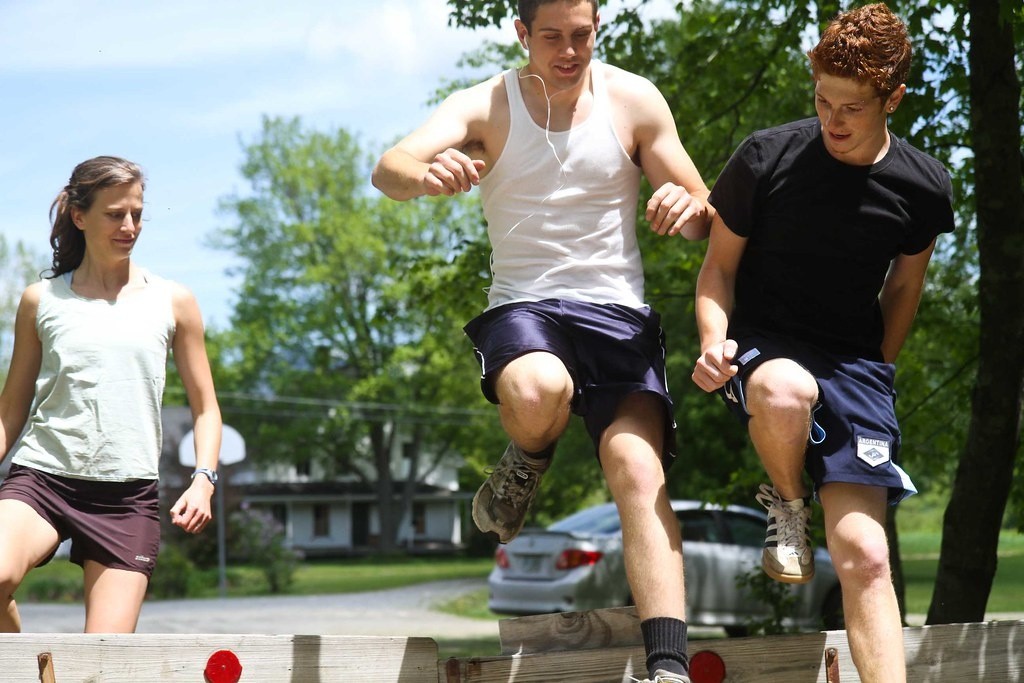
[191,469,218,486]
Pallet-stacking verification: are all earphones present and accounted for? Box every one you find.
[523,35,530,51]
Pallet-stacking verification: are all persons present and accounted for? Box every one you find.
[372,0,716,683]
[693,3,955,683]
[0,156,223,634]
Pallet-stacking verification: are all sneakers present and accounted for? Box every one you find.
[472,434,559,543]
[631,668,692,682]
[756,483,814,583]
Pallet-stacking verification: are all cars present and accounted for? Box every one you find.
[487,497,846,639]
